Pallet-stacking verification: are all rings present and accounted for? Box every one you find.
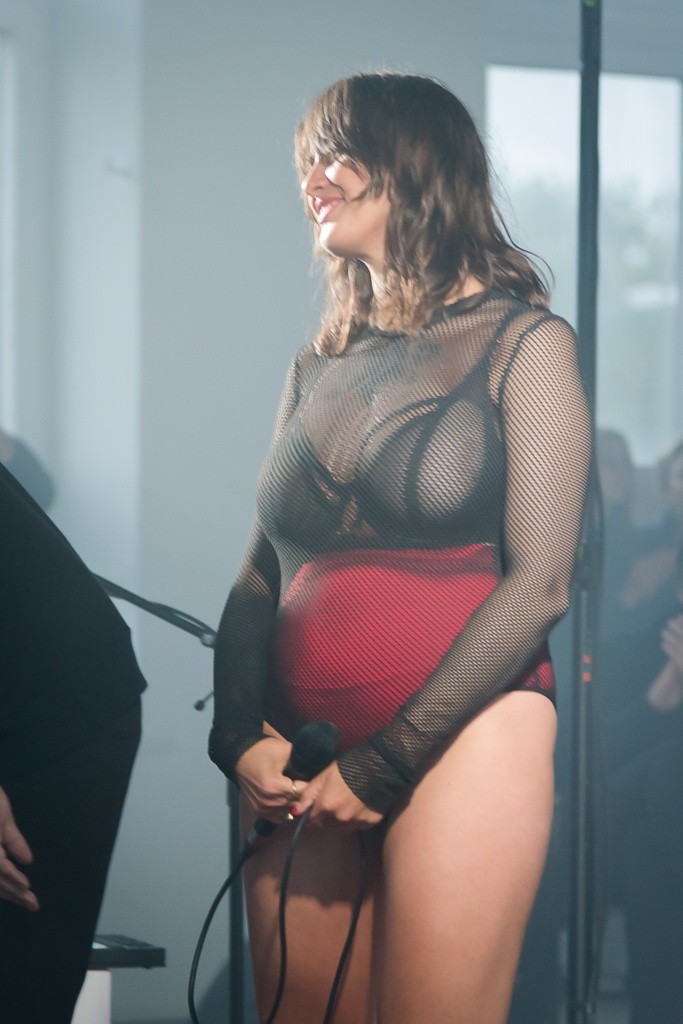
[284,778,300,801]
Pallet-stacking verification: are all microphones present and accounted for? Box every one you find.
[239,718,337,862]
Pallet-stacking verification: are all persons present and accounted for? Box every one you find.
[514,422,683,1024]
[0,462,147,1024]
[207,72,594,1023]
